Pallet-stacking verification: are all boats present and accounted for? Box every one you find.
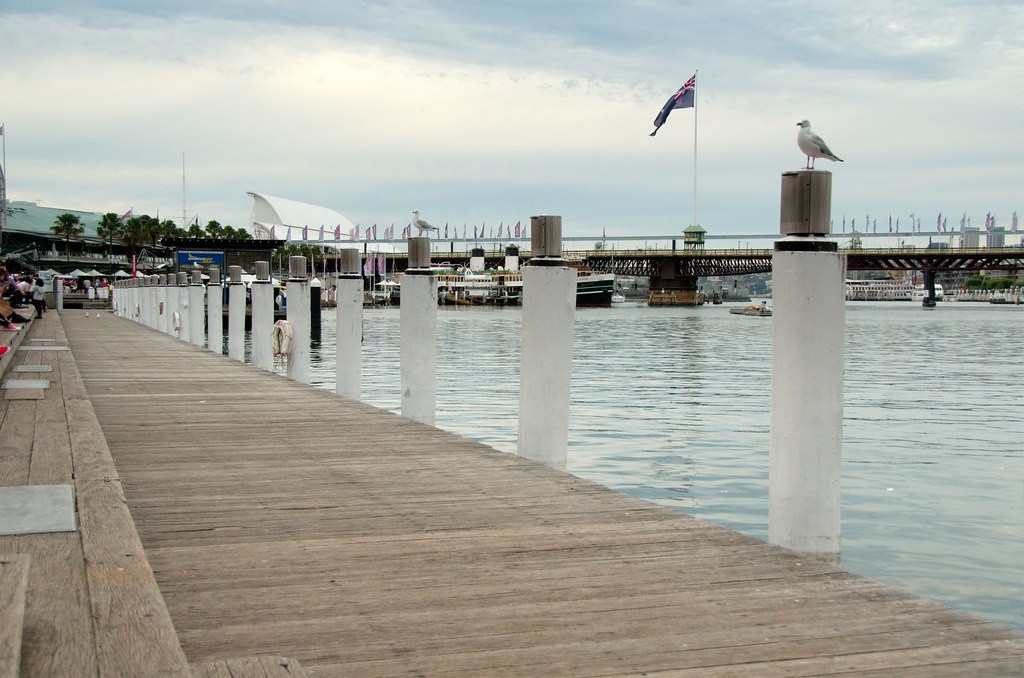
[911,282,944,302]
[728,300,773,317]
[204,262,626,330]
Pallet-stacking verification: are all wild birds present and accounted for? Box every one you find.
[412,209,439,236]
[85,312,90,318]
[96,313,100,320]
[796,119,844,169]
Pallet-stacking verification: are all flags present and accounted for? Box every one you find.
[384,225,394,240]
[445,223,466,239]
[364,255,385,275]
[1011,213,1016,229]
[302,224,359,241]
[937,213,946,233]
[651,75,695,135]
[985,213,994,231]
[120,209,132,221]
[473,223,484,239]
[889,214,899,233]
[960,216,970,232]
[498,222,526,238]
[909,213,920,232]
[287,227,291,240]
[366,224,376,240]
[402,224,422,240]
[269,226,275,240]
[831,214,876,233]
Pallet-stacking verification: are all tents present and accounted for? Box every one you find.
[46,269,149,285]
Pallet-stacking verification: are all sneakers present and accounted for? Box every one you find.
[0,345,11,357]
[3,322,21,330]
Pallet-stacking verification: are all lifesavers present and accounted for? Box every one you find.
[465,269,473,278]
[275,295,282,305]
[272,319,292,357]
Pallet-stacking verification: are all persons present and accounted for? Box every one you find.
[61,278,109,288]
[0,264,45,356]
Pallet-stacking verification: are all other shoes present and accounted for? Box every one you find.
[11,314,31,323]
[35,315,43,319]
